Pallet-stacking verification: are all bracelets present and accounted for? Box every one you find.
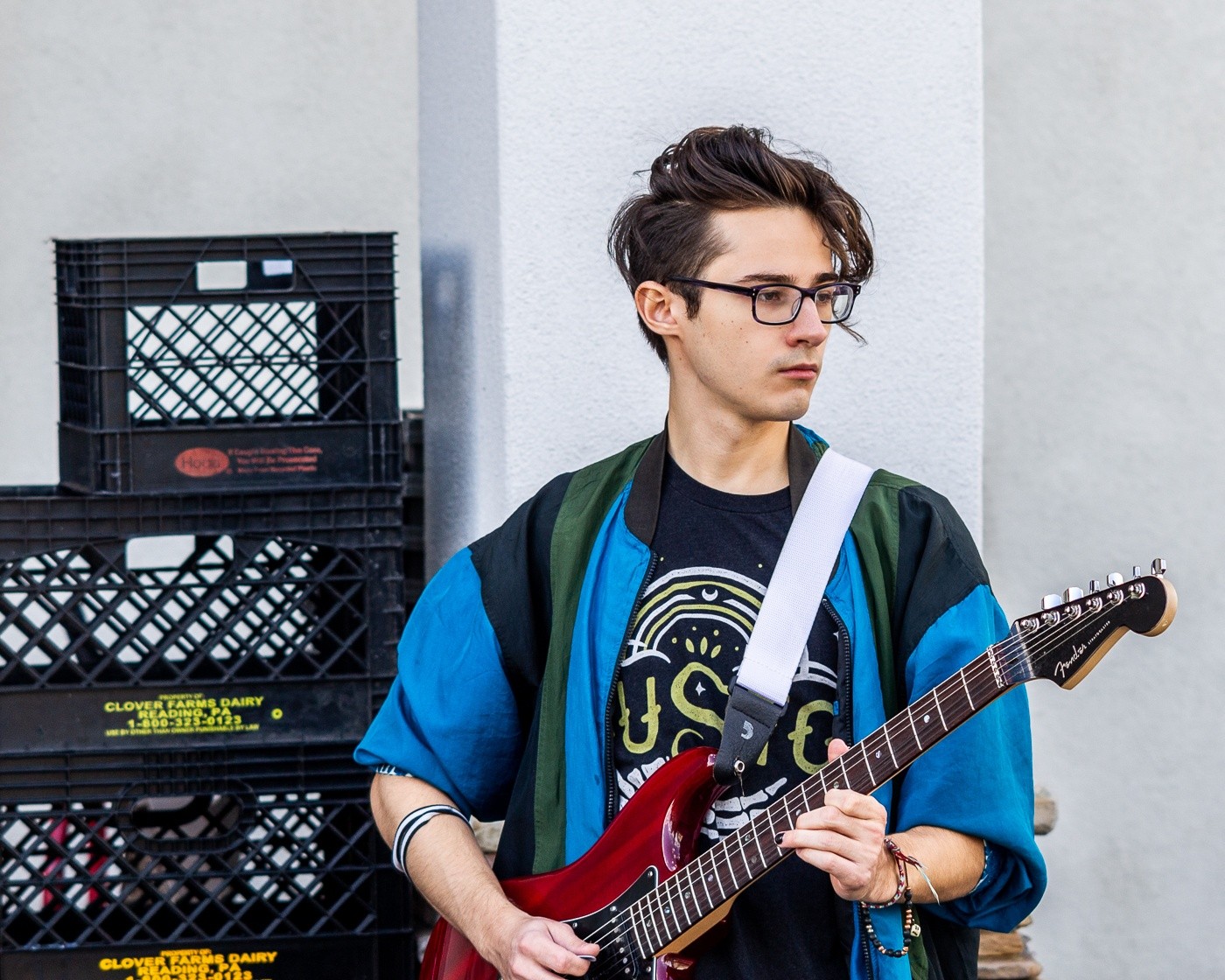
[390,801,478,882]
[857,836,921,958]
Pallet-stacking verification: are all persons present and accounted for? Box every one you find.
[351,126,1048,980]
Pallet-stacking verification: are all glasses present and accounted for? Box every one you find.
[661,275,861,326]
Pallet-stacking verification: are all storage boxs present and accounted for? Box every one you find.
[0,482,415,980]
[52,233,407,494]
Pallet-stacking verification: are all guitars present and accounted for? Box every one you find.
[420,557,1177,980]
